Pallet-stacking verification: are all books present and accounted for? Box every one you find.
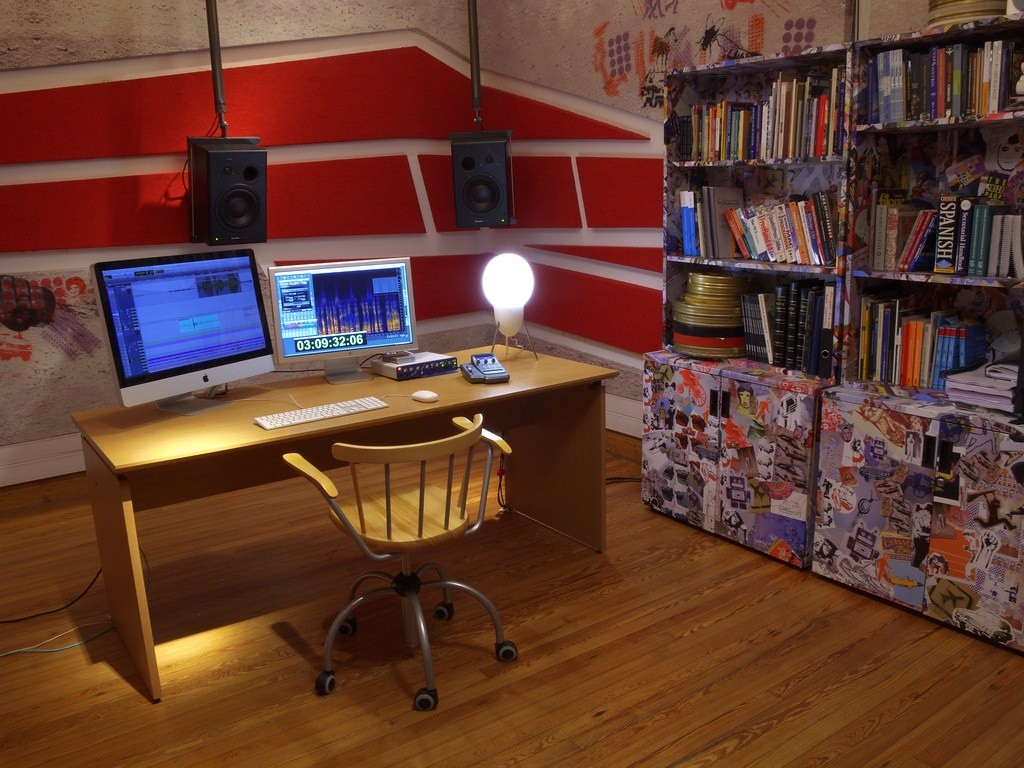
[738,276,835,380]
[863,38,1024,126]
[677,183,838,268]
[852,288,1022,418]
[690,67,848,163]
[866,186,1024,282]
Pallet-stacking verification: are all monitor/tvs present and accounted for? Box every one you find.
[269,256,419,385]
[89,248,276,416]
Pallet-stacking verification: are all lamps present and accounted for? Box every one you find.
[482,251,537,363]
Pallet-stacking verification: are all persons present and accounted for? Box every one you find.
[983,121,1023,175]
[64,276,90,318]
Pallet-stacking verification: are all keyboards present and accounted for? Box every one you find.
[253,396,389,430]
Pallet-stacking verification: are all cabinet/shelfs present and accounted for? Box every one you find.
[640,42,854,570]
[810,21,1024,658]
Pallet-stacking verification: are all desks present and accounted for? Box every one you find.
[71,345,621,702]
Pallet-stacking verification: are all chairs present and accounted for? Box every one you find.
[283,413,517,710]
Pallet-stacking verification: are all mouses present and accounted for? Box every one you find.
[412,390,438,402]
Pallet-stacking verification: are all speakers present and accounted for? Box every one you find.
[450,138,511,228]
[190,142,267,247]
